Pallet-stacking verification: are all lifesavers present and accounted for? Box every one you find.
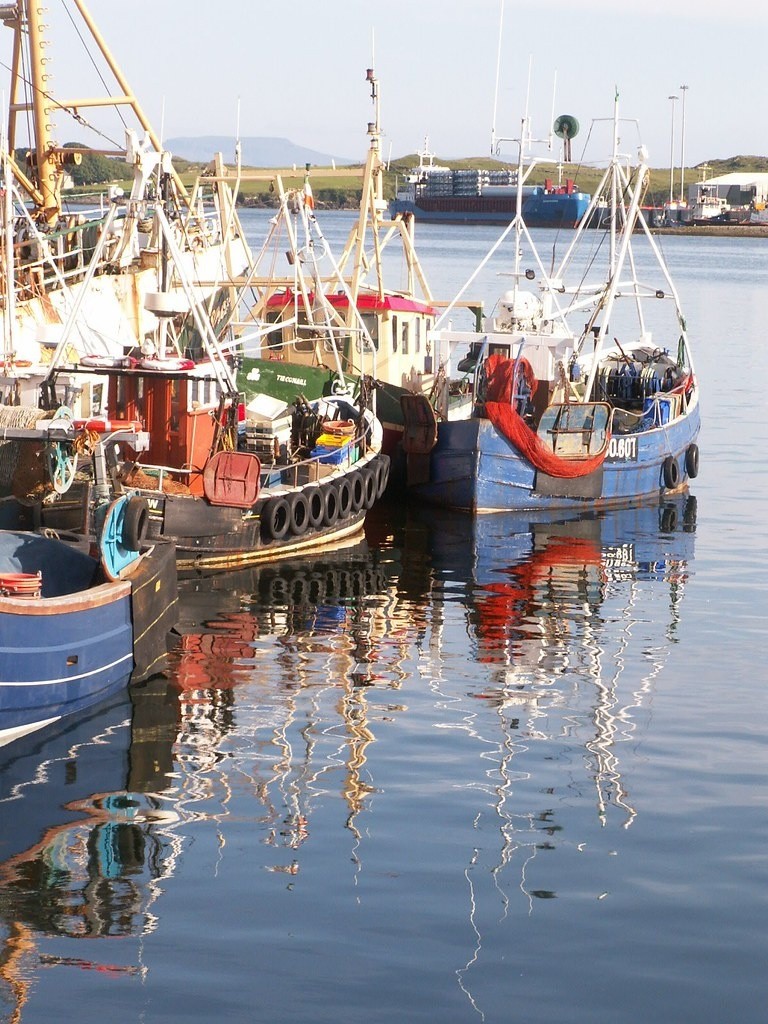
[72,420,143,432]
[369,459,385,499]
[301,486,325,528]
[80,354,139,369]
[261,497,291,541]
[378,454,390,490]
[331,476,352,518]
[687,444,699,479]
[359,467,377,509]
[121,496,149,551]
[683,495,697,532]
[665,456,680,489]
[139,356,195,371]
[347,471,364,512]
[0,359,33,367]
[662,503,679,534]
[319,483,339,527]
[285,491,309,536]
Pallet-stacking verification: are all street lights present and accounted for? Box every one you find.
[679,85,689,201]
[668,96,679,201]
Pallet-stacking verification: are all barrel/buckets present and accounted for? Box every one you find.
[321,418,356,441]
[0,570,42,600]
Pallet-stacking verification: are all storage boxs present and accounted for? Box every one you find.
[643,391,682,427]
[726,211,751,222]
[244,415,351,488]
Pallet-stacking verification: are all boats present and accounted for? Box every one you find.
[1,407,181,747]
[389,136,591,230]
[0,0,450,572]
[167,534,433,887]
[398,1,701,515]
[421,490,698,1023]
[0,671,178,1024]
